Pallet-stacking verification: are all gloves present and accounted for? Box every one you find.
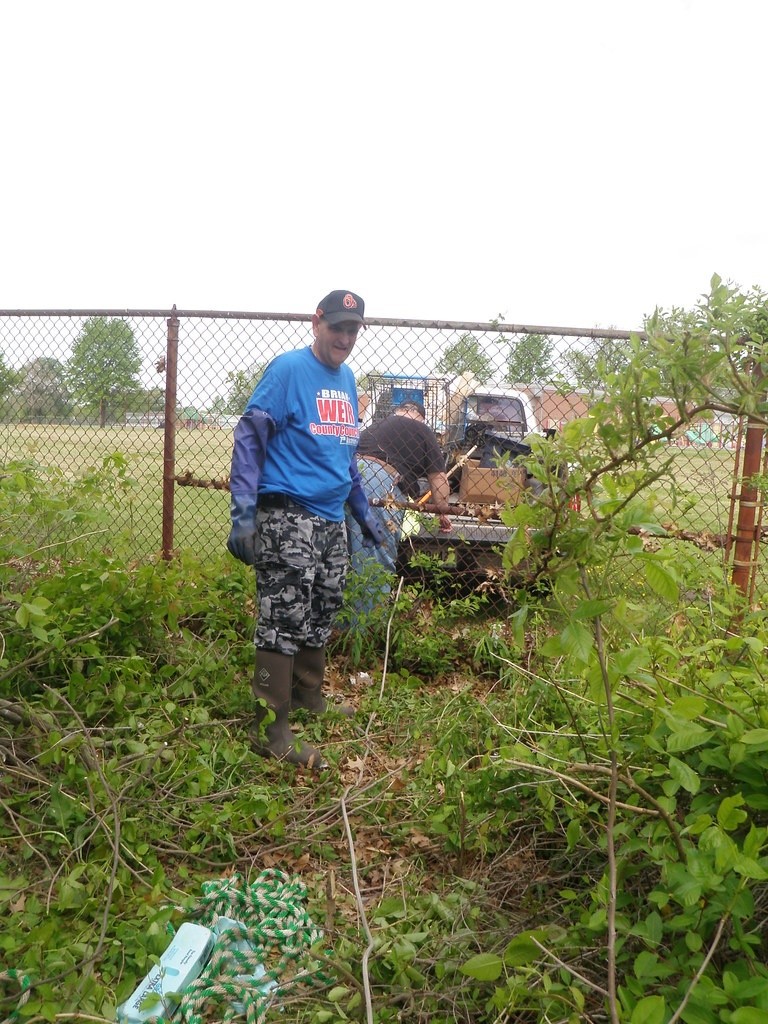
[227,409,277,566]
[346,439,384,549]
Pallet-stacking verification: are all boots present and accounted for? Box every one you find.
[246,645,355,767]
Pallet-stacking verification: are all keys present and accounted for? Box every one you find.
[390,483,396,493]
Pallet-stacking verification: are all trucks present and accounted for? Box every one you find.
[360,384,581,598]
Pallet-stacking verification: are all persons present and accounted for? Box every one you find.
[226,290,387,767]
[343,402,452,636]
[435,432,444,454]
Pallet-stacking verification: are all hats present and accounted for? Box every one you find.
[316,290,367,331]
[400,400,425,418]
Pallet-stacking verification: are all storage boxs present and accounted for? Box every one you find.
[459,459,526,505]
[464,549,529,578]
[478,430,532,468]
